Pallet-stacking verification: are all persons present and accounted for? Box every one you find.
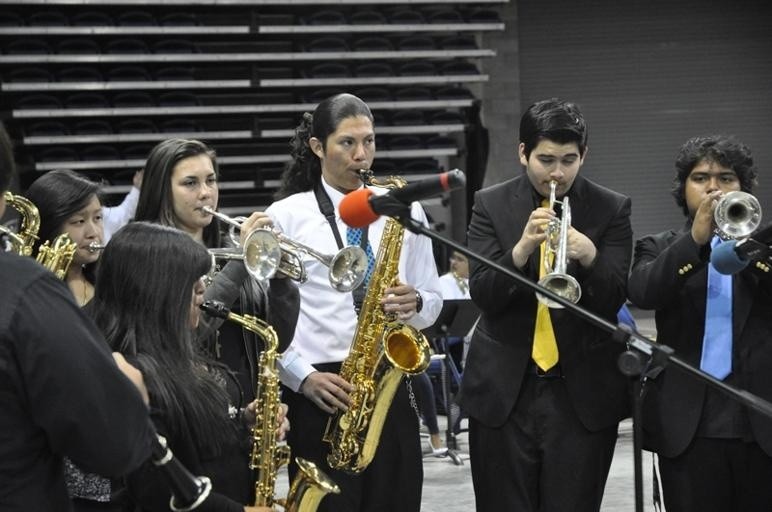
[454,97,633,512]
[83,222,290,512]
[435,247,472,303]
[626,134,772,512]
[410,368,449,459]
[100,168,144,252]
[132,138,301,512]
[17,168,106,316]
[0,121,149,512]
[245,93,444,512]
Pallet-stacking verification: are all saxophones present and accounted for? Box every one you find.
[323,166,431,476]
[1,192,76,279]
[200,300,341,512]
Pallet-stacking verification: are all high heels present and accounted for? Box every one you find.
[428,437,449,458]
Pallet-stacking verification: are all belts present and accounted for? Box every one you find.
[526,365,565,381]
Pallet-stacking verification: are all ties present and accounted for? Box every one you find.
[344,226,375,289]
[531,197,560,373]
[699,235,733,383]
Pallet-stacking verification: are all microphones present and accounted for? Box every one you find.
[710,223,772,275]
[337,168,468,229]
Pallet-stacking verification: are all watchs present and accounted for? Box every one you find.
[413,288,423,314]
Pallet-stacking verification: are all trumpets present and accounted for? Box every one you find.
[205,205,368,293]
[711,189,763,237]
[89,229,281,280]
[537,182,583,310]
